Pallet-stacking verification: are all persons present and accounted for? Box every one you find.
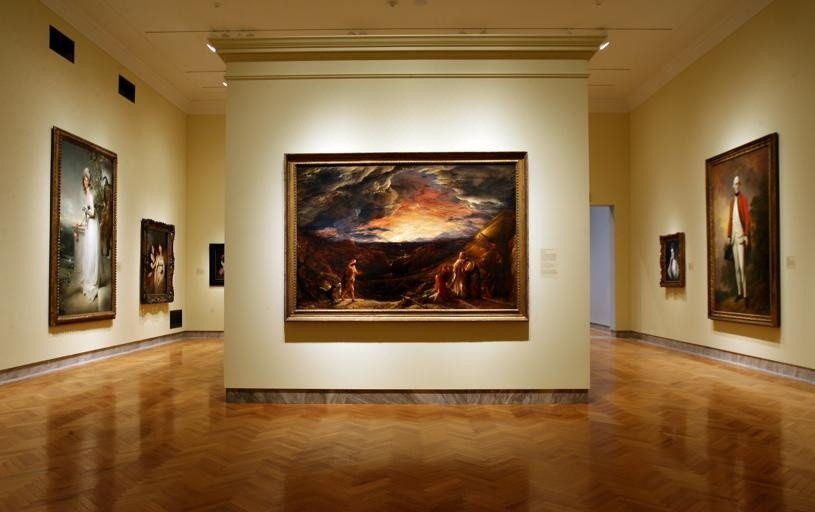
[74,167,101,304]
[428,274,445,303]
[340,259,362,302]
[446,251,470,299]
[146,243,156,294]
[667,242,679,280]
[727,176,750,309]
[154,243,166,293]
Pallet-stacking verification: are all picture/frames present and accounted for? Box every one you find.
[660,232,685,287]
[209,243,224,286]
[704,132,781,328]
[141,218,175,303]
[49,125,117,328]
[283,153,529,324]
